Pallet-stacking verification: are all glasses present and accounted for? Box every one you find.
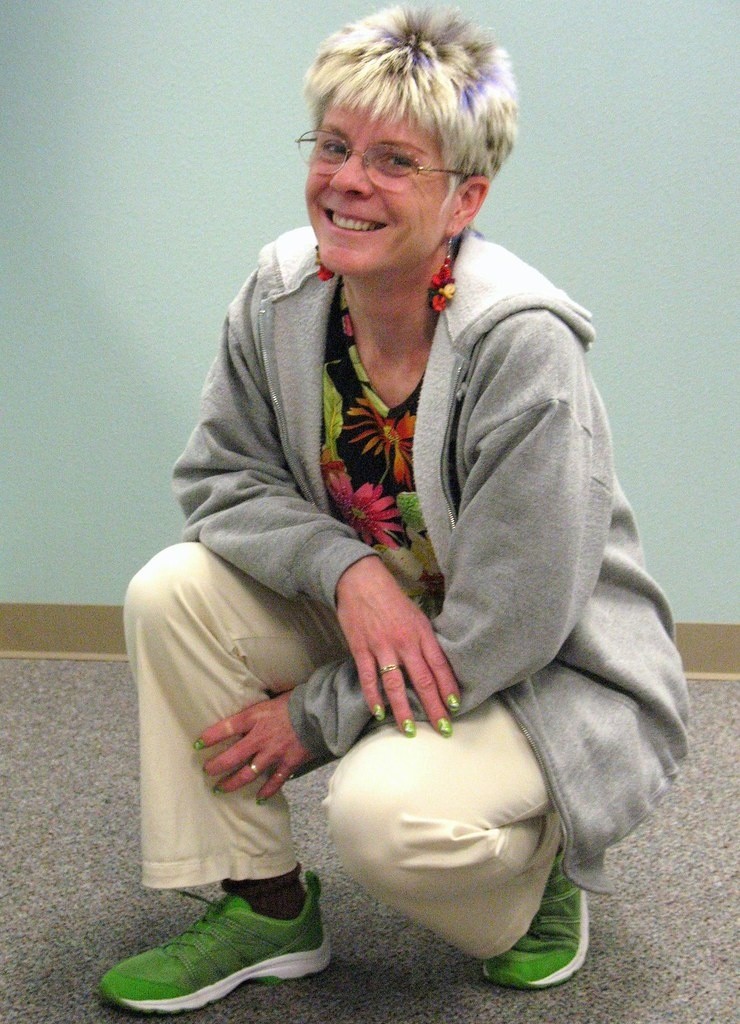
[292,129,474,191]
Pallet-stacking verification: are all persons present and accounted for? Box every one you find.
[94,1,689,1015]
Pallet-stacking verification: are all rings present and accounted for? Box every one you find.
[250,764,258,774]
[276,772,285,778]
[379,665,401,674]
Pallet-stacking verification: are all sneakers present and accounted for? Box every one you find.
[480,846,591,990]
[97,869,333,1014]
